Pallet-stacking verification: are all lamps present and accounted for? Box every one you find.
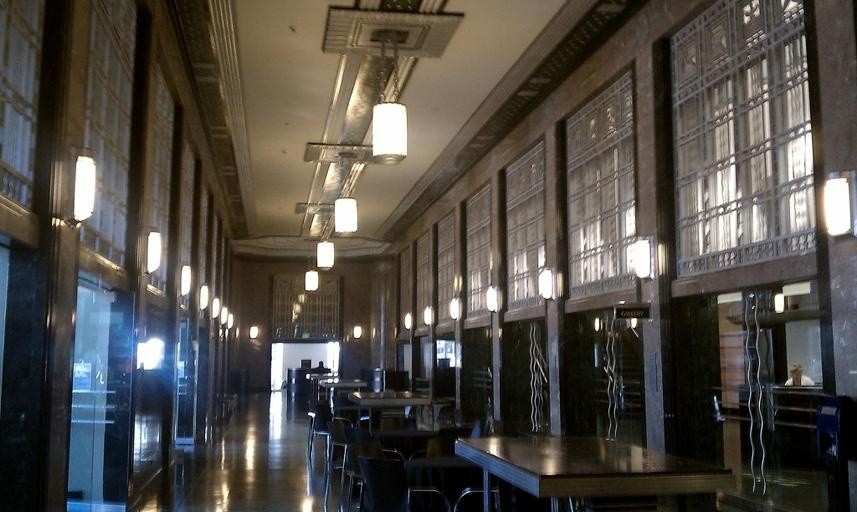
[65,148,262,343]
[132,335,167,374]
[815,168,853,240]
[627,235,668,283]
[284,164,359,326]
[369,32,411,161]
[405,285,503,331]
[536,265,565,300]
